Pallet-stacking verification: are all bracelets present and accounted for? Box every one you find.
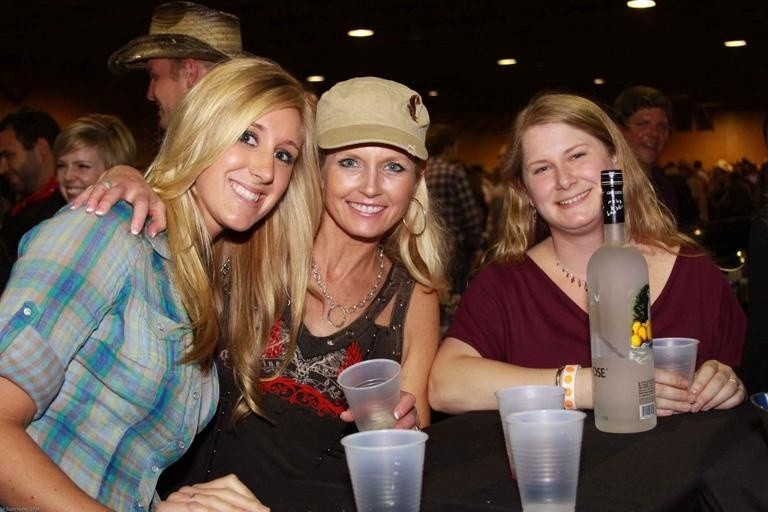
[554,363,581,411]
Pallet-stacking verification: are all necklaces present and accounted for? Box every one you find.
[309,249,385,327]
[554,256,588,292]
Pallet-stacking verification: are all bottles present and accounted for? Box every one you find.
[587,170,658,434]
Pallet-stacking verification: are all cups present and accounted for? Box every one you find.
[336,358,401,434]
[340,429,429,512]
[652,337,700,385]
[505,409,587,512]
[494,385,566,479]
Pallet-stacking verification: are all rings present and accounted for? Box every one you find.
[729,378,740,387]
[100,177,112,190]
[189,492,198,501]
[737,385,747,394]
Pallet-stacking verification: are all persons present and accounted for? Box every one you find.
[0,56,324,512]
[106,0,242,147]
[68,76,440,433]
[52,113,136,202]
[613,85,701,237]
[427,124,480,238]
[654,156,768,248]
[0,107,67,296]
[427,93,747,418]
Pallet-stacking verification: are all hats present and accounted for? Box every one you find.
[314,76,431,162]
[107,1,258,74]
